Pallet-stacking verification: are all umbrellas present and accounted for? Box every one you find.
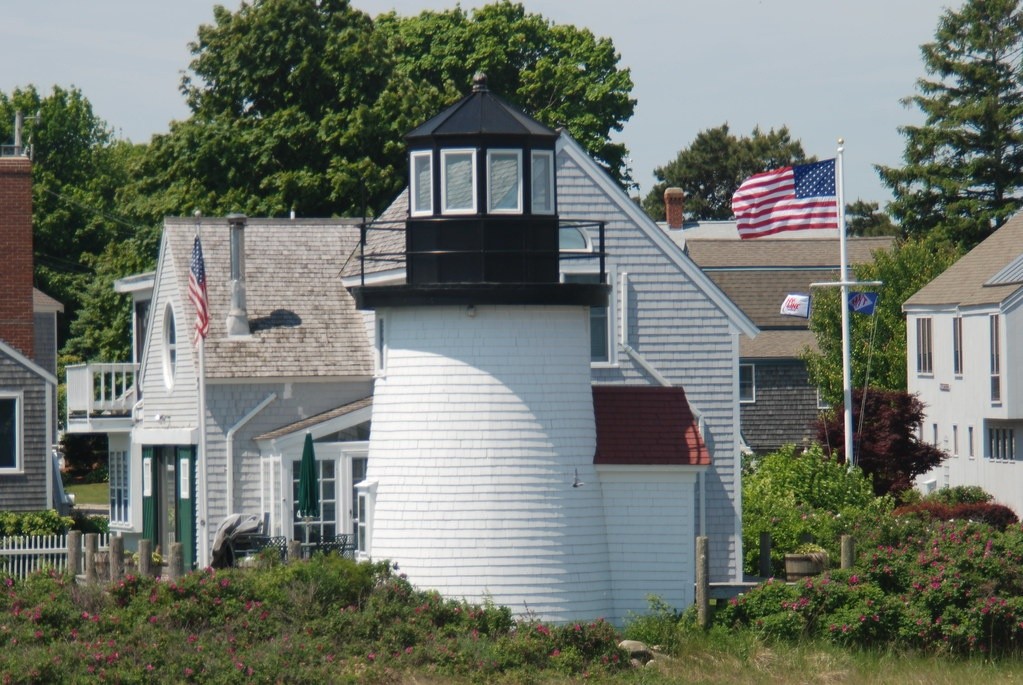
[298,431,319,559]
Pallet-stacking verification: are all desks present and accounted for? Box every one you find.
[298,542,339,559]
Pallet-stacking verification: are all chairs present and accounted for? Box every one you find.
[250,532,357,562]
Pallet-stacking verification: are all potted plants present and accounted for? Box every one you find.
[784,541,830,581]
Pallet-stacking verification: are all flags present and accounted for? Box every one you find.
[731,157,838,240]
[848,292,878,315]
[779,294,811,319]
[186,235,212,346]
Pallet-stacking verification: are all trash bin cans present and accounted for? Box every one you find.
[782,554,824,583]
